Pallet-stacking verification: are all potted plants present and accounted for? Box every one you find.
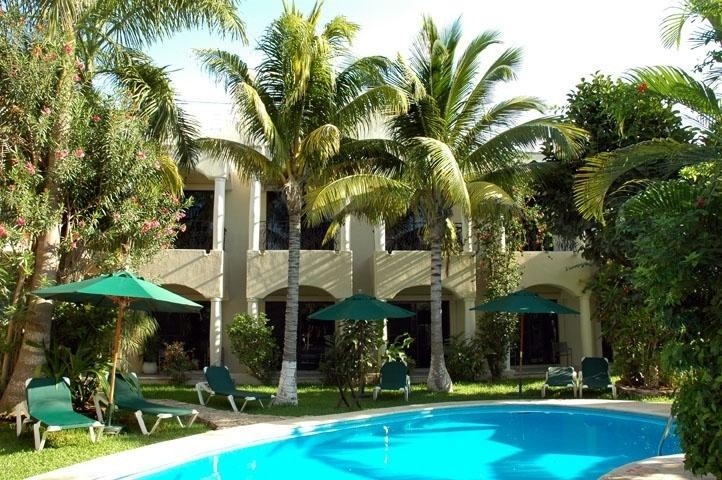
[445,330,466,382]
[142,349,158,375]
[319,333,349,385]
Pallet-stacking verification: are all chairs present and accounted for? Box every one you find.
[553,342,572,366]
[195,366,275,413]
[541,367,577,398]
[578,356,617,398]
[373,362,410,401]
[93,372,200,435]
[16,377,105,450]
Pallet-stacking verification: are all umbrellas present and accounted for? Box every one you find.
[32,264,205,427]
[468,287,581,392]
[306,288,416,393]
